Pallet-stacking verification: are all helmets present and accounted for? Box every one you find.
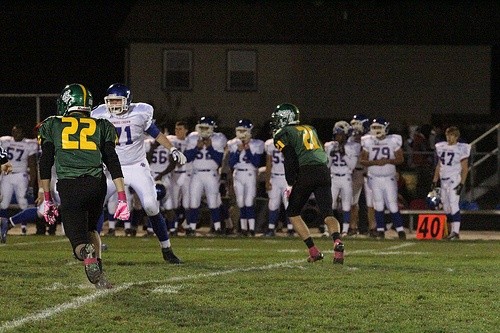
[333,121,351,136]
[195,117,215,140]
[426,190,443,210]
[351,113,369,135]
[370,117,389,140]
[235,118,253,142]
[270,102,300,138]
[56,83,94,116]
[104,85,132,117]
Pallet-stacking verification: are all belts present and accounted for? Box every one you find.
[153,168,287,178]
[332,172,450,182]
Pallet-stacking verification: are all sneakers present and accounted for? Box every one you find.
[169,224,298,239]
[446,232,460,241]
[104,227,154,238]
[94,273,113,289]
[307,252,325,262]
[0,218,12,244]
[83,243,99,283]
[321,229,406,241]
[20,226,65,236]
[332,239,344,264]
[162,253,180,264]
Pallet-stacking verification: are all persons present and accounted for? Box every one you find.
[90,84,187,275]
[346,113,371,235]
[264,111,302,239]
[359,116,407,242]
[320,120,361,240]
[426,125,471,240]
[227,117,264,239]
[37,83,132,285]
[271,101,345,267]
[0,116,235,245]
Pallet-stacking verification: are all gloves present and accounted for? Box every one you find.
[431,182,437,190]
[43,192,59,225]
[453,183,463,195]
[169,146,187,165]
[114,191,130,221]
